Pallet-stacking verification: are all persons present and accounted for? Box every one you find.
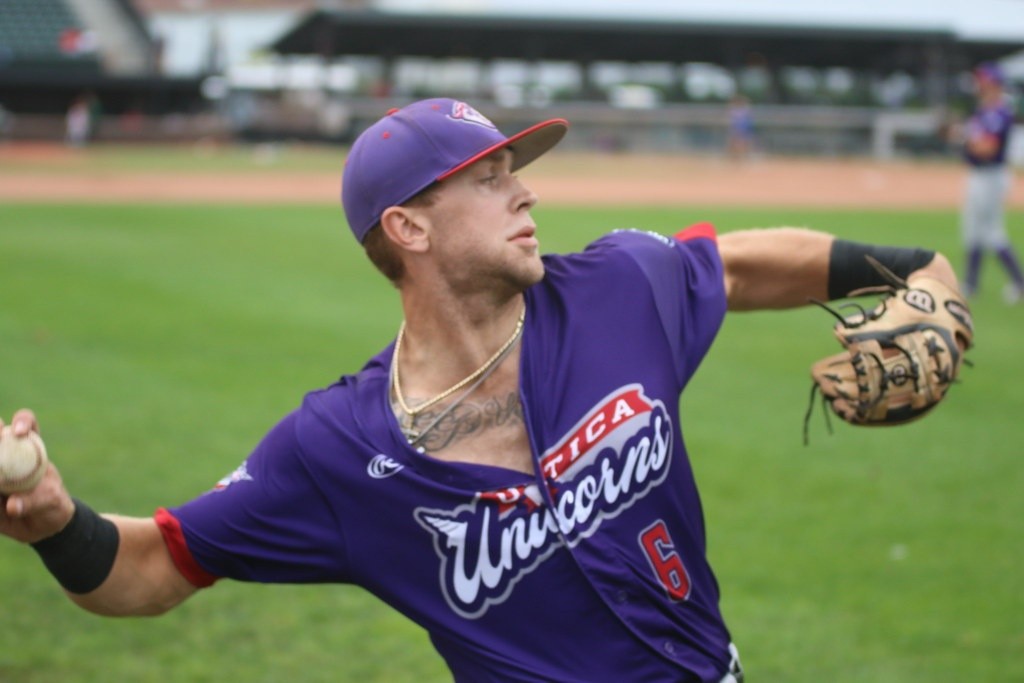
[945,61,1024,307]
[0,97,975,683]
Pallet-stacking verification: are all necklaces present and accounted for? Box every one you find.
[390,330,523,445]
[393,306,525,415]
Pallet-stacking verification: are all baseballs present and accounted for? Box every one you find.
[0,424,46,493]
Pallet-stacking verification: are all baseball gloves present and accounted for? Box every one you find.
[799,250,975,447]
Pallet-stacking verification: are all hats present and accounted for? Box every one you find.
[340,98,569,244]
